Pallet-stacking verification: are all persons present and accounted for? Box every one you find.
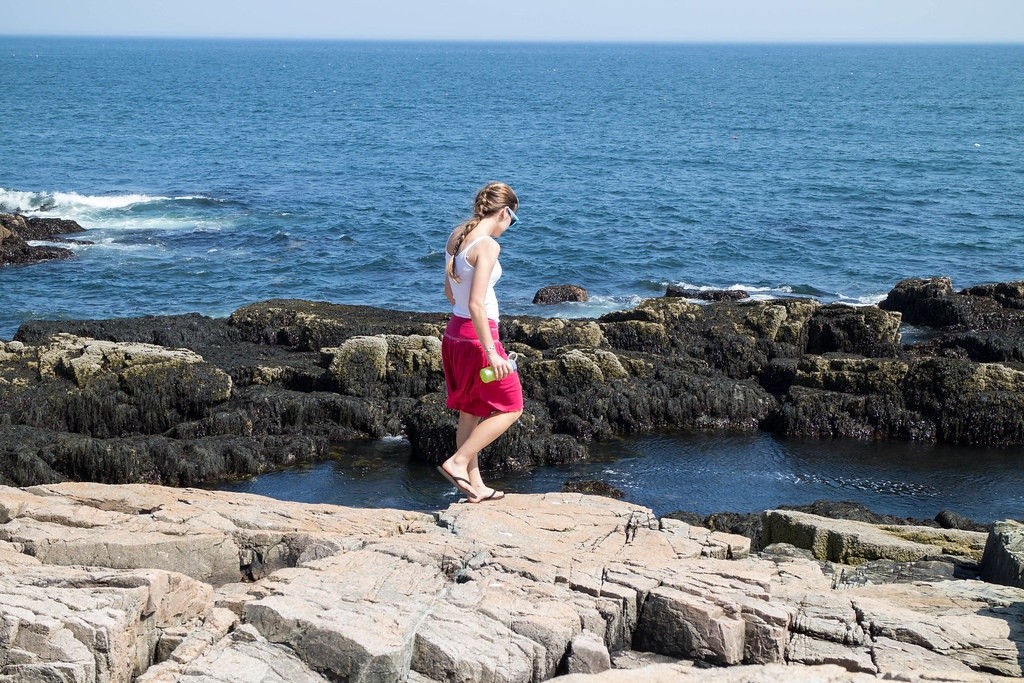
[436,182,525,503]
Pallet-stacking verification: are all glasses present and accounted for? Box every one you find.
[509,208,519,227]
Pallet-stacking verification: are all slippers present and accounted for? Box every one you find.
[465,490,504,503]
[437,466,477,499]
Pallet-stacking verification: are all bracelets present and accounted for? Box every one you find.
[485,343,495,352]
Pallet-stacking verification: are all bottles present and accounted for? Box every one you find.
[480,352,518,383]
